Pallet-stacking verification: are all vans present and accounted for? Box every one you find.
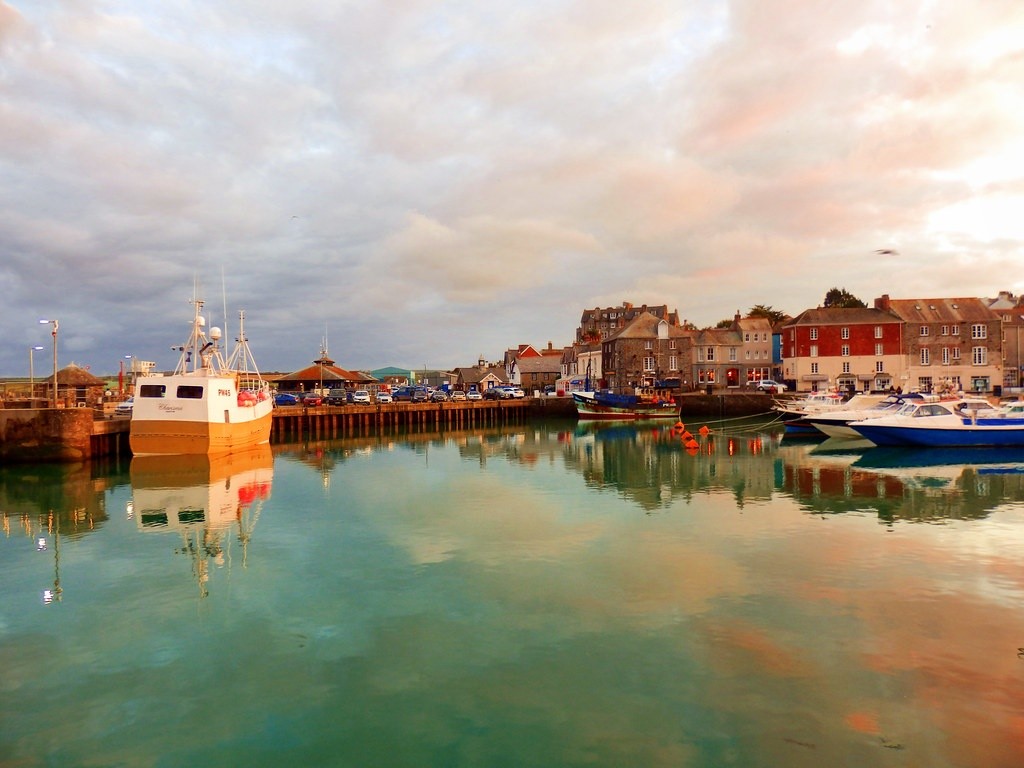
[393,387,428,401]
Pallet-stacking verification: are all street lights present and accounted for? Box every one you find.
[125,355,137,381]
[40,320,59,407]
[30,346,44,398]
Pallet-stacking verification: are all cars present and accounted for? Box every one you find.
[303,392,321,407]
[374,392,393,404]
[482,388,509,400]
[450,390,466,402]
[294,392,329,403]
[412,389,427,403]
[273,393,299,406]
[116,396,133,414]
[427,390,448,402]
[466,391,483,401]
[328,388,347,405]
[346,391,371,405]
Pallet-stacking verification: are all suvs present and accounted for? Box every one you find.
[757,380,788,391]
[504,388,525,399]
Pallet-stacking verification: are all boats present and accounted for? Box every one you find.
[129,296,274,457]
[572,388,683,417]
[129,445,274,598]
[770,392,1024,447]
